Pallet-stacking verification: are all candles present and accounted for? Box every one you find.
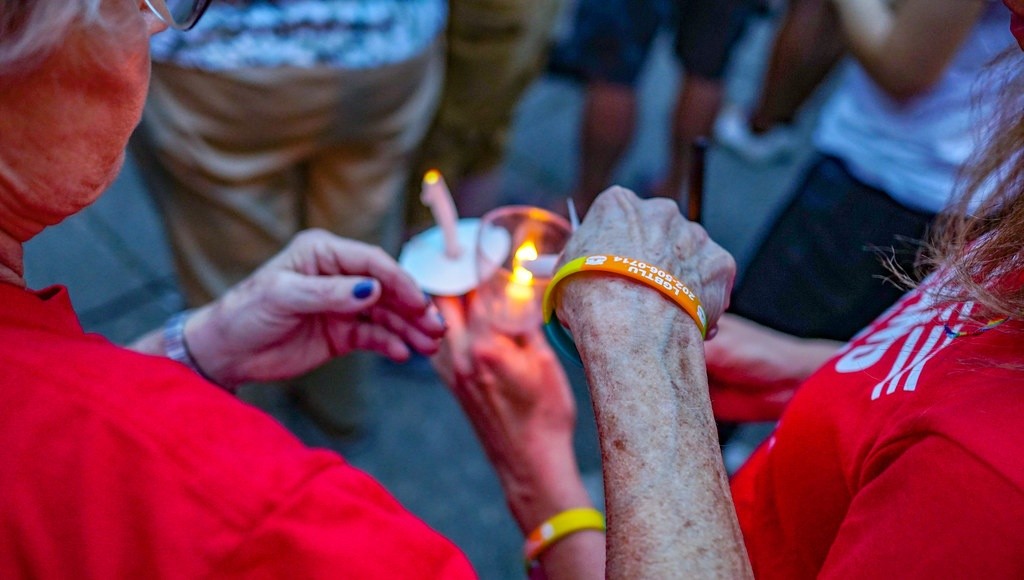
[420,169,466,260]
[503,240,539,321]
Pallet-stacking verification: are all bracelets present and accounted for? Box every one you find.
[162,309,239,396]
[523,507,606,573]
[543,255,707,370]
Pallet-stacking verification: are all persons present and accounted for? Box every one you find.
[0,0,1024,580]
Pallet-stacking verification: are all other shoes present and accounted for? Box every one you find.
[273,378,372,444]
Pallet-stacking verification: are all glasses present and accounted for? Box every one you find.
[144,0,213,32]
